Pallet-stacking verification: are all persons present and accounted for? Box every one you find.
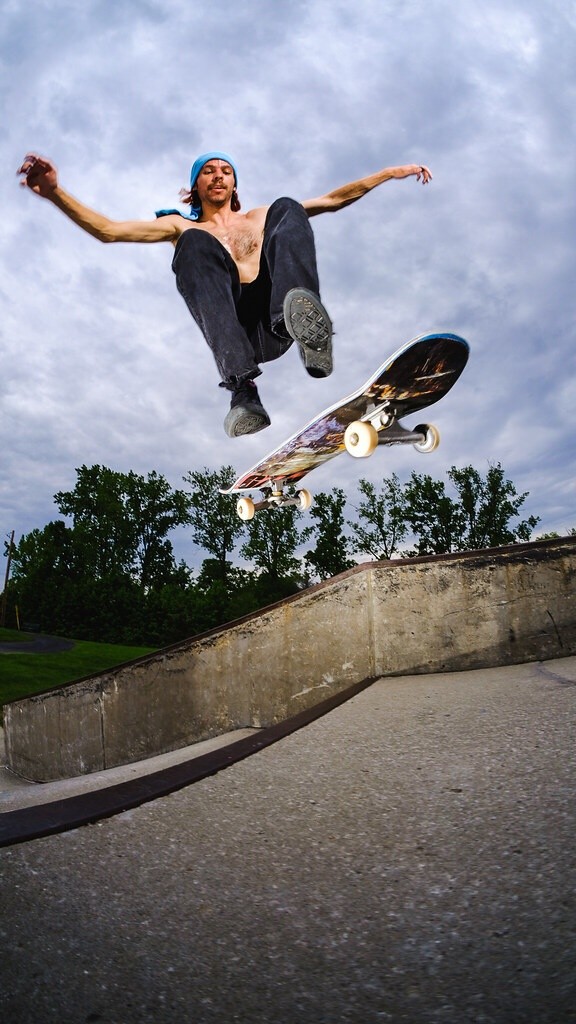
[17,152,431,435]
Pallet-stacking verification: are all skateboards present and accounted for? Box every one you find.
[217,329,471,521]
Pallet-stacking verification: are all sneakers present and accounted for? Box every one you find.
[283,287,336,378]
[223,379,271,439]
